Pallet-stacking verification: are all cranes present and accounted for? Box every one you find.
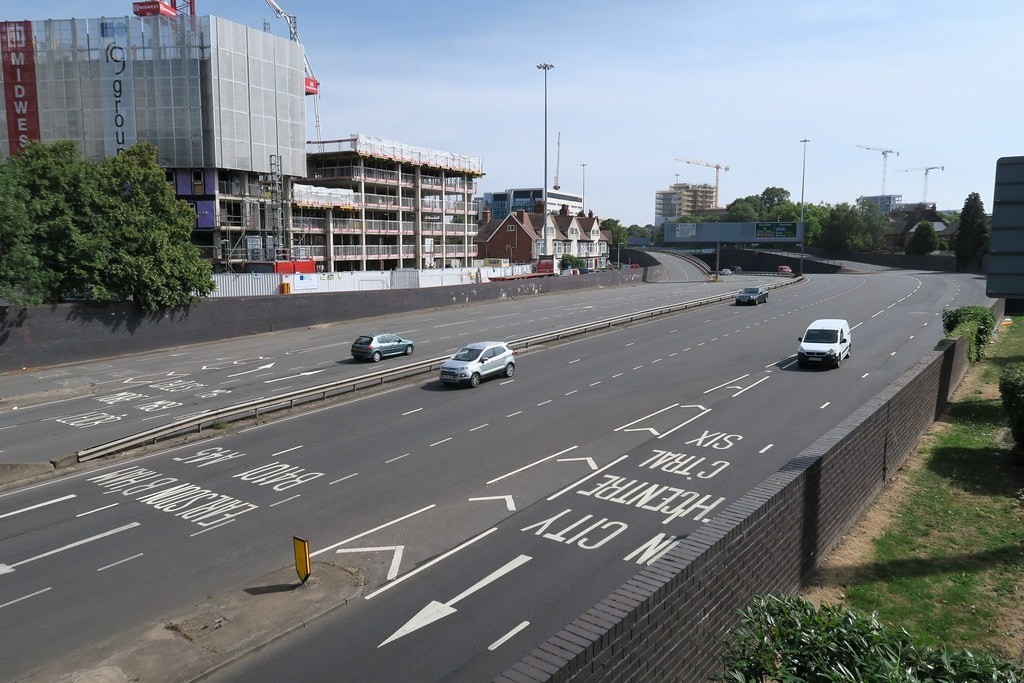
[672,156,728,207]
[553,131,561,191]
[854,144,901,204]
[902,165,945,208]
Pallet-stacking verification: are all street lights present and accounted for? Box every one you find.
[537,62,555,254]
[799,138,812,260]
[674,173,681,183]
[580,163,588,214]
[617,242,624,270]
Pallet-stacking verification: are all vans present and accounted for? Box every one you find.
[778,265,792,273]
[796,318,852,368]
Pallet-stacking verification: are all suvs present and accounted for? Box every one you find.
[440,340,516,389]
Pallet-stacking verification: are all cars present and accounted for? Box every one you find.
[719,269,732,276]
[735,287,769,305]
[350,332,416,362]
[730,266,742,272]
